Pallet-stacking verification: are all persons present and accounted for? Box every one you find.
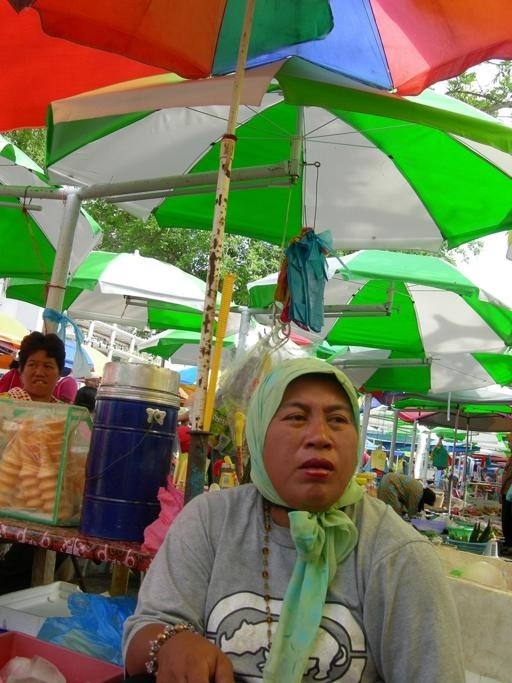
[375,471,437,519]
[115,355,466,683]
[498,432,512,557]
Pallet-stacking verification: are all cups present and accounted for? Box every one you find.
[490,542,498,558]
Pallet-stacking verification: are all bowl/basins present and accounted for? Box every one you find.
[411,519,447,534]
[53,376,78,404]
[446,527,488,555]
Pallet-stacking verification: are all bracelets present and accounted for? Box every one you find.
[145,623,200,676]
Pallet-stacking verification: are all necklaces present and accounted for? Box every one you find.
[262,501,273,652]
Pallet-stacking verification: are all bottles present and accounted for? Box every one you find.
[210,400,235,438]
[218,463,239,488]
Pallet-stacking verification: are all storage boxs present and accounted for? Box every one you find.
[0,631,125,682]
[1,580,84,637]
[1,397,93,527]
[439,546,512,683]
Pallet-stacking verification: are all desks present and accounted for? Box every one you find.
[1,513,159,596]
